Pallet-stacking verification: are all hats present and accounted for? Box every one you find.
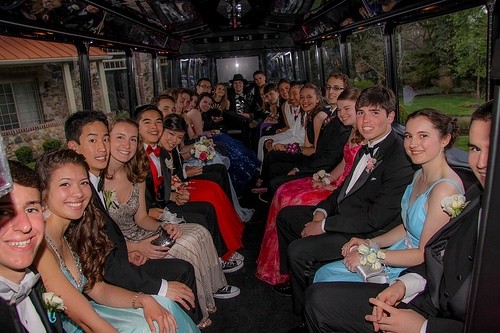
[229,74,248,84]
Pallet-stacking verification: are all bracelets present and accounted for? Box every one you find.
[278,128,283,133]
[312,169,337,185]
[159,207,187,231]
[286,142,303,155]
[366,238,373,246]
[357,242,386,269]
[132,292,144,309]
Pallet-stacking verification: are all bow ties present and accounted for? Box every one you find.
[365,147,374,157]
[10,273,41,305]
[146,145,161,157]
[97,171,106,192]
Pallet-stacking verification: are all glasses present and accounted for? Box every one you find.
[199,85,212,89]
[324,86,347,91]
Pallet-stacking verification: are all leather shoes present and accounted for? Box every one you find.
[288,321,307,333]
[272,280,292,296]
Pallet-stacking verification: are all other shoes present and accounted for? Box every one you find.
[229,251,246,261]
[212,284,241,300]
[215,257,245,273]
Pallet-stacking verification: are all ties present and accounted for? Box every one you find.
[321,111,332,128]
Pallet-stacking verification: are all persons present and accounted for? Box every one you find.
[0,159,68,333]
[31,148,204,333]
[152,64,329,224]
[100,116,228,330]
[261,72,350,204]
[304,100,499,333]
[271,86,416,333]
[313,108,465,286]
[131,104,244,299]
[255,86,367,284]
[65,110,208,332]
[153,114,247,262]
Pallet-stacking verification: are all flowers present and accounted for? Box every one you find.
[42,292,66,323]
[322,116,333,130]
[249,120,260,129]
[158,207,187,226]
[190,136,216,168]
[441,194,471,219]
[206,130,220,138]
[286,142,304,156]
[365,157,377,173]
[103,188,119,211]
[357,244,387,271]
[211,116,223,123]
[170,176,190,207]
[164,158,175,170]
